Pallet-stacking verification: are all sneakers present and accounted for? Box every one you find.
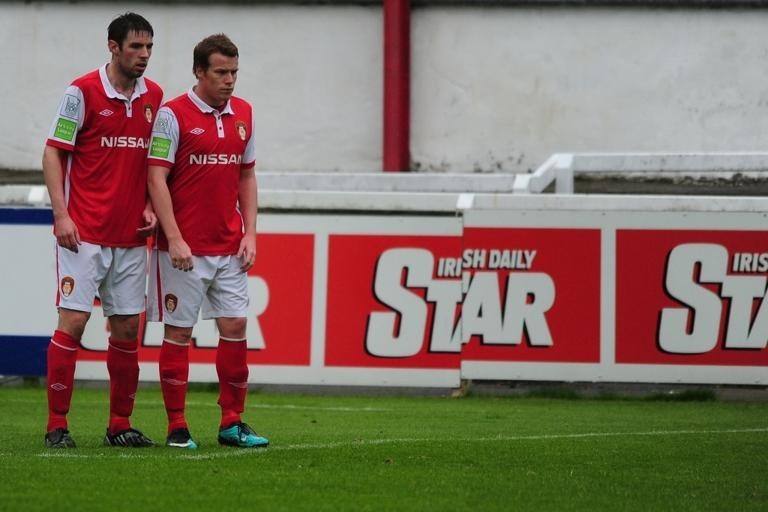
[218,422,269,447]
[104,427,155,447]
[45,427,75,448]
[166,428,197,449]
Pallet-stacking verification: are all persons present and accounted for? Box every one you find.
[145,33,272,450]
[41,12,156,451]
